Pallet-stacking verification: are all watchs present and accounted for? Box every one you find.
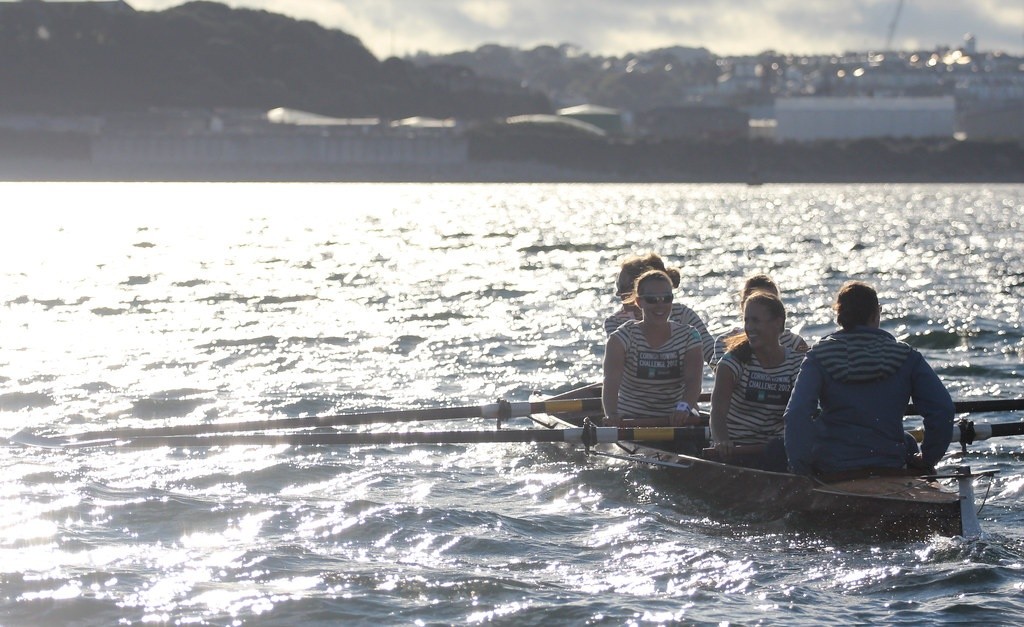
[675,402,694,417]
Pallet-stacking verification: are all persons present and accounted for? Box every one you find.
[602,269,703,428]
[780,280,955,480]
[714,274,808,380]
[604,252,720,373]
[708,291,807,474]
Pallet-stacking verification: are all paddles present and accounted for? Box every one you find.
[908,400,1024,485]
[58,381,703,452]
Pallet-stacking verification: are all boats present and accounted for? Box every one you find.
[525,379,983,542]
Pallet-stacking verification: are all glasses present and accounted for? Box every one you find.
[615,280,631,292]
[639,293,674,304]
